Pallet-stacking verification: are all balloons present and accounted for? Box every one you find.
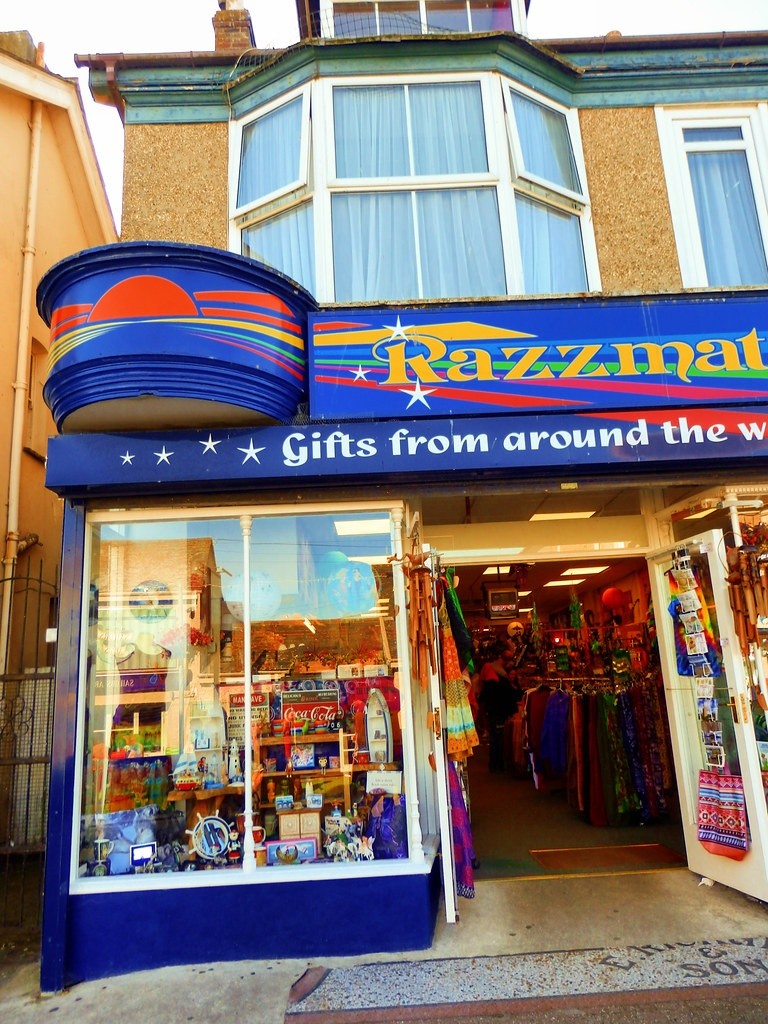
[600,586,624,609]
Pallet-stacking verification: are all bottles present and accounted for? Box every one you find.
[280,777,289,796]
[294,777,301,802]
[267,777,276,803]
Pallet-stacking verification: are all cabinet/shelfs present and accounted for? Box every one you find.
[108,727,395,841]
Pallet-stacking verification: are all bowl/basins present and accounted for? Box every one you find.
[35,242,319,435]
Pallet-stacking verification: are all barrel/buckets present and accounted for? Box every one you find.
[236,812,259,834]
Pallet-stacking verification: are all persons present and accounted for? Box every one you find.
[104,632,171,808]
[468,639,541,770]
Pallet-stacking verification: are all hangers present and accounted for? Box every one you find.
[525,676,644,696]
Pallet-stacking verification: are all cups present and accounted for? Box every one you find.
[352,751,370,765]
[314,721,328,734]
[252,826,266,845]
[273,720,284,737]
[329,756,340,769]
[255,847,267,867]
[291,720,304,736]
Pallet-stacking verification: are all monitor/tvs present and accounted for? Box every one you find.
[488,589,519,616]
